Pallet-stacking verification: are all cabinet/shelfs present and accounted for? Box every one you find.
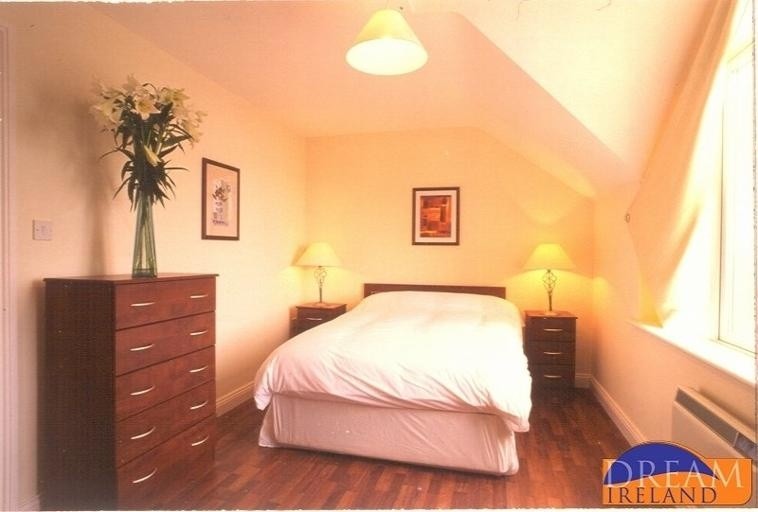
[42,272,217,511]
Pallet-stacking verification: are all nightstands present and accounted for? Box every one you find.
[296,302,347,335]
[522,310,579,394]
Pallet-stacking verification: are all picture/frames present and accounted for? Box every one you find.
[201,157,240,240]
[411,186,460,245]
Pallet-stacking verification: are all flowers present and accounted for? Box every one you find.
[88,74,210,277]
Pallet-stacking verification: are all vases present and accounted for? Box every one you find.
[132,183,158,279]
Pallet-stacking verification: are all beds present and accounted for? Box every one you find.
[270,283,520,477]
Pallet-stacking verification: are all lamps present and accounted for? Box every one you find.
[522,243,577,317]
[346,5,429,76]
[294,241,343,306]
